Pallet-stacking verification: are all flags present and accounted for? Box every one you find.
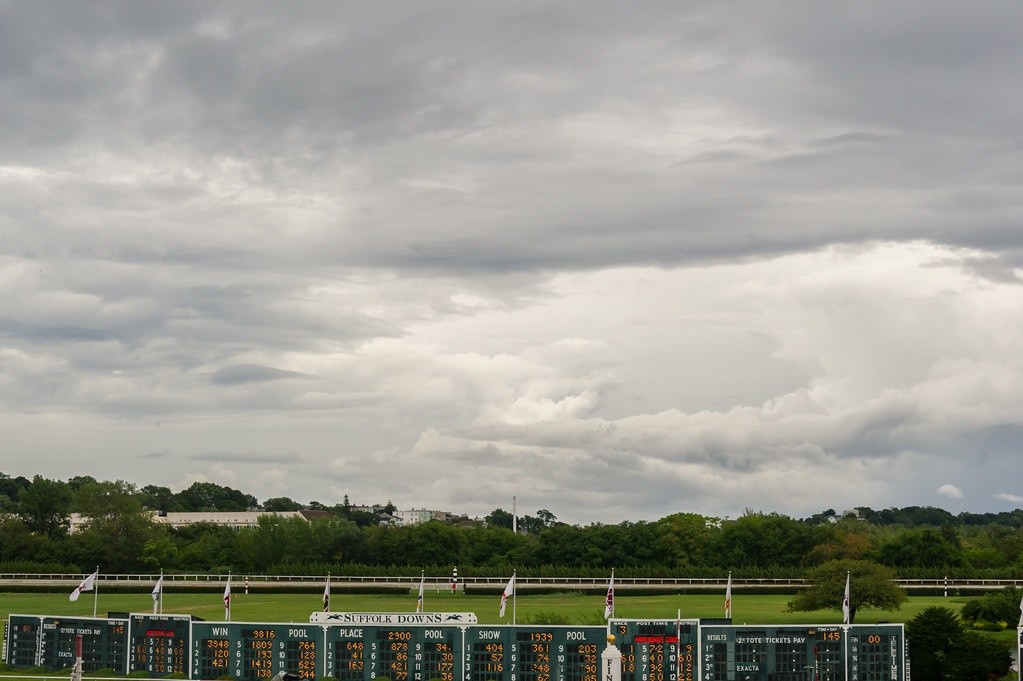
[322,577,330,612]
[416,580,422,613]
[604,572,614,620]
[69,572,95,603]
[151,577,161,613]
[725,574,732,618]
[499,574,514,618]
[223,577,229,621]
[842,575,849,623]
